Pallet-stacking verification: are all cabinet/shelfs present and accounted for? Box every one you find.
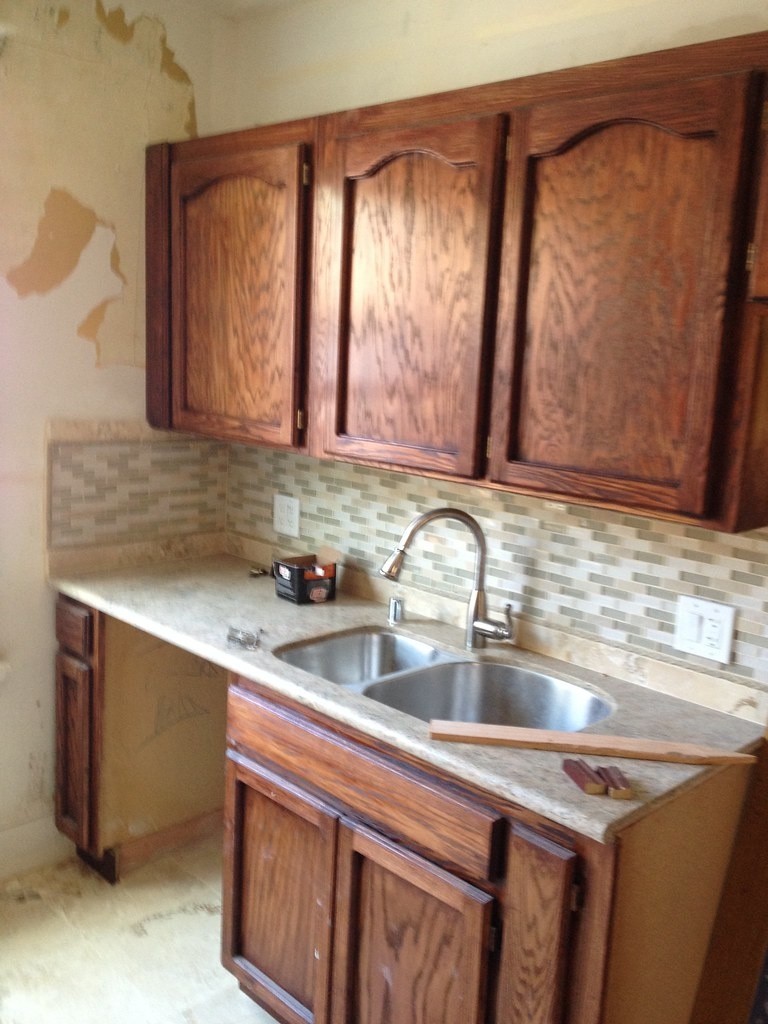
[53,591,227,886]
[170,142,311,448]
[221,674,768,1024]
[321,68,759,519]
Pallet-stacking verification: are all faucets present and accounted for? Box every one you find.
[378,506,519,649]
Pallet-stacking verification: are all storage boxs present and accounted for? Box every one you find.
[273,544,342,606]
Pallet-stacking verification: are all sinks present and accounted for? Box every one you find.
[361,660,615,733]
[272,628,444,689]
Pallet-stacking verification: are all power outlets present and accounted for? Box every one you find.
[674,595,736,665]
[272,494,300,538]
